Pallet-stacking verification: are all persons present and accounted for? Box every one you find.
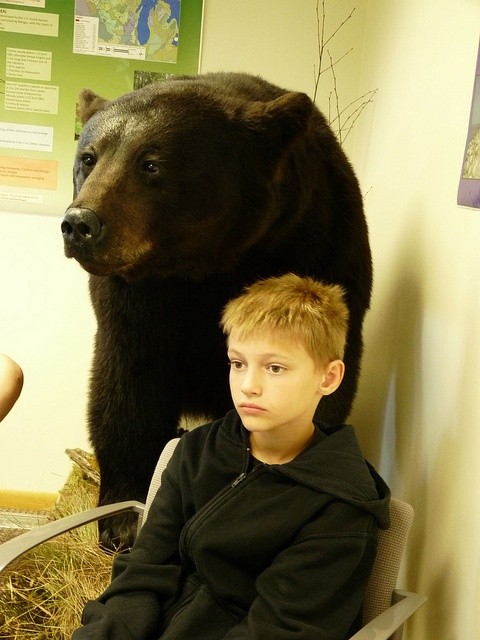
[70,272,393,640]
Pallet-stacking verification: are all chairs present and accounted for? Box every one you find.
[0,437,427,640]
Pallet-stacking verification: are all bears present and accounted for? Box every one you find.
[62,72,373,551]
